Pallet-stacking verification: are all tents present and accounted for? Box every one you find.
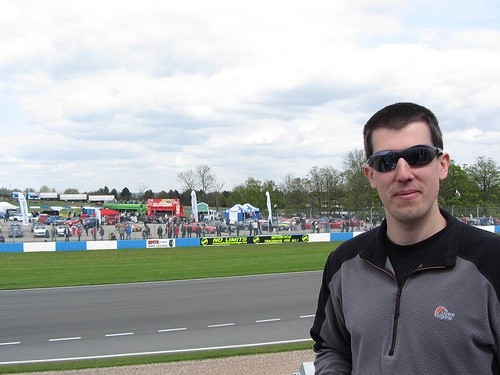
[242,202,259,222]
[223,204,251,224]
[101,209,120,225]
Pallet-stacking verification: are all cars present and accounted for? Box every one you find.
[54,225,72,236]
[34,226,48,238]
[2,209,367,238]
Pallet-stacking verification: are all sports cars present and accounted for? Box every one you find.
[183,222,216,234]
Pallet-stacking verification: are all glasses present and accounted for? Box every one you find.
[362,144,443,173]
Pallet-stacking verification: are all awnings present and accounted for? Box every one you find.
[107,203,145,220]
[147,198,181,218]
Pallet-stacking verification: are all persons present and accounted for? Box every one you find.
[309,102,500,375]
[0,208,500,243]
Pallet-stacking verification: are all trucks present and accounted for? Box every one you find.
[11,189,116,204]
